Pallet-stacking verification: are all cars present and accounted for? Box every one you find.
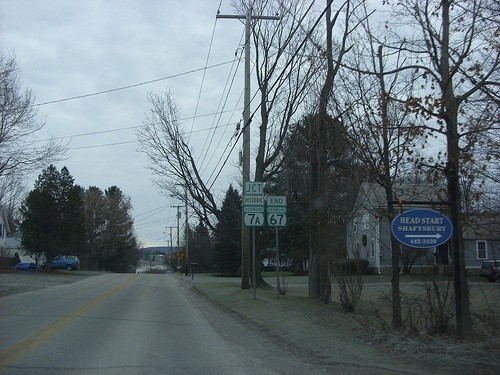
[15,262,35,270]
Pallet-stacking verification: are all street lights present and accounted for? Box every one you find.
[170,193,189,276]
[163,232,172,269]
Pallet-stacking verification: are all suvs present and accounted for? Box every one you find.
[479,260,500,283]
[41,255,80,271]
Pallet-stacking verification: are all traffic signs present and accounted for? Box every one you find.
[391,207,454,249]
